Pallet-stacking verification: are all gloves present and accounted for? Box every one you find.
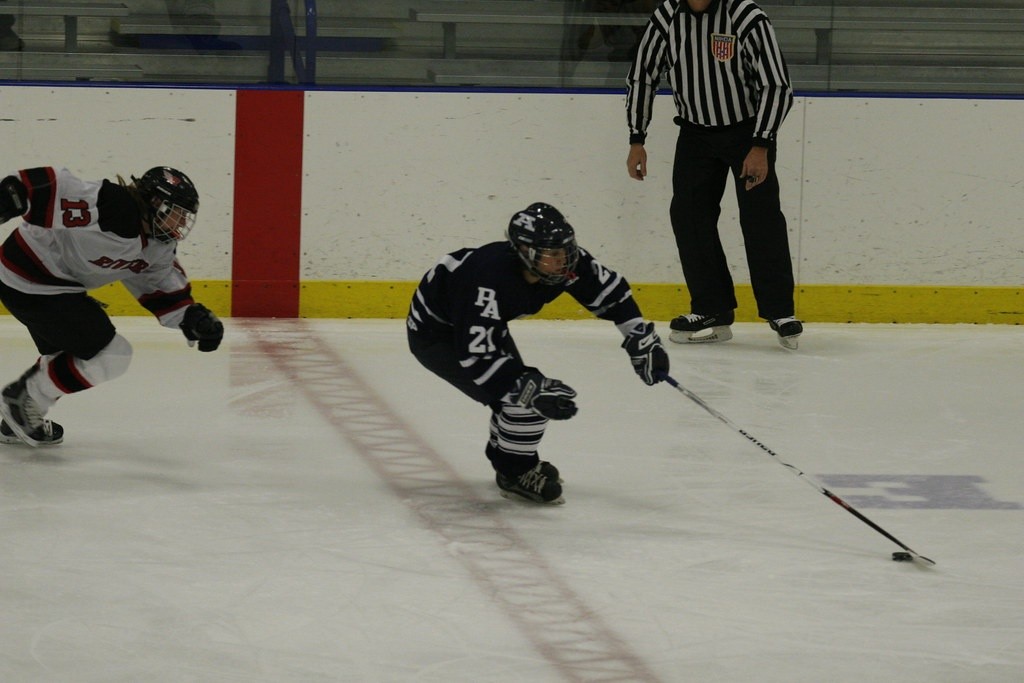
[510,371,578,420]
[0,174,29,225]
[179,302,225,352]
[622,322,670,386]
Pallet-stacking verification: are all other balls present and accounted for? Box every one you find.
[892,552,911,561]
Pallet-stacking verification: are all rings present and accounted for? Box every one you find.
[746,175,758,183]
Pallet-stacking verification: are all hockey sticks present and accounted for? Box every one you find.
[659,369,936,566]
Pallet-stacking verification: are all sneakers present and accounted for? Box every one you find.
[767,316,803,350]
[495,470,565,507]
[0,362,53,448]
[0,417,64,449]
[492,459,564,484]
[669,309,734,344]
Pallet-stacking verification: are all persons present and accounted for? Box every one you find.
[0,166,224,448]
[623,0,805,350]
[405,202,669,505]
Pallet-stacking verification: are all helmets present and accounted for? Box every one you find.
[508,201,579,285]
[131,166,198,246]
